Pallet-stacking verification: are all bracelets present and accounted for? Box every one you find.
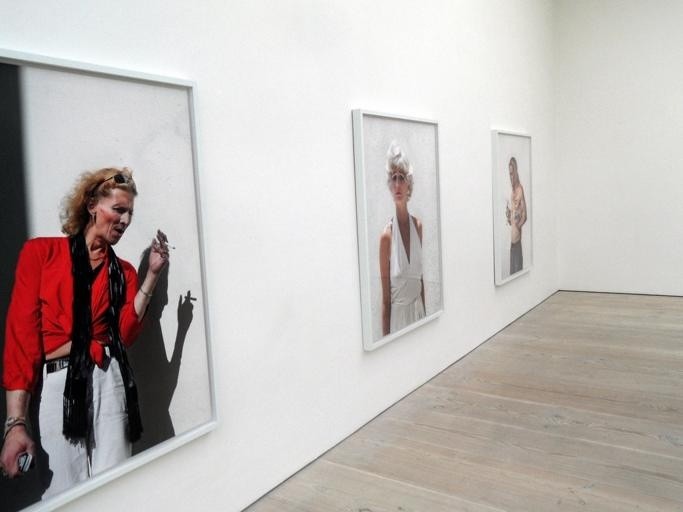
[138,286,154,297]
[2,416,29,441]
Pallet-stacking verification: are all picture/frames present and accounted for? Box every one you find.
[0,50,220,512]
[490,127,535,289]
[351,104,445,354]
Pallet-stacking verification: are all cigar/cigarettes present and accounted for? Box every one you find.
[165,241,176,250]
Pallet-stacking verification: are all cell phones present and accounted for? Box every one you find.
[18,453,33,473]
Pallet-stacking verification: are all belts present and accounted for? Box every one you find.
[46,357,70,372]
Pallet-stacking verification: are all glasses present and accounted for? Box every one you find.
[102,173,125,183]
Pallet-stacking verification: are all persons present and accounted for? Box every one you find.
[505,156,527,276]
[0,168,169,499]
[378,140,428,335]
